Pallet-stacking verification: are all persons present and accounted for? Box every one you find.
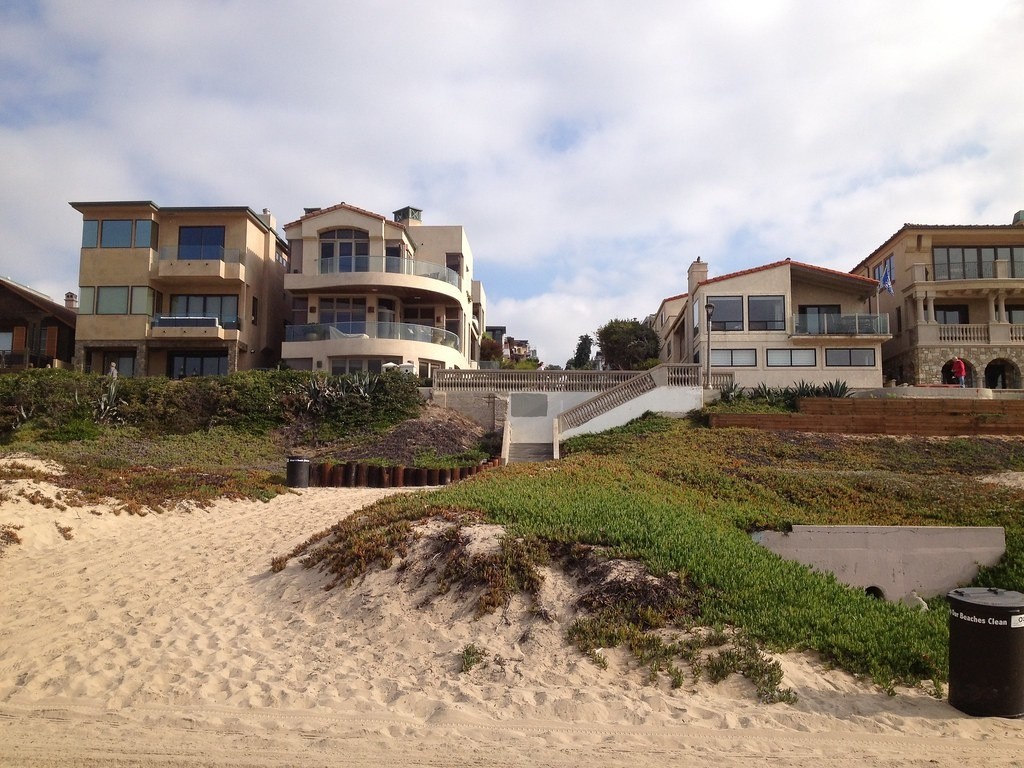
[951,356,965,388]
[537,362,545,380]
[107,362,118,381]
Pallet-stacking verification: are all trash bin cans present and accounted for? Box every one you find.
[944,586,1024,719]
[286,455,310,488]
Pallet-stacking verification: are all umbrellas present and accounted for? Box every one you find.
[382,362,399,369]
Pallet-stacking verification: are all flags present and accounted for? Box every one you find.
[882,265,894,297]
